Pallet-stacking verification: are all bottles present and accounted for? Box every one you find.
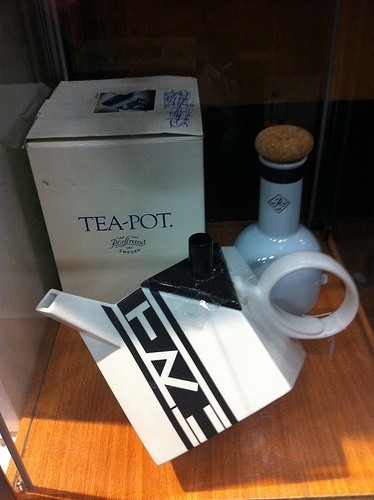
[234,124,321,315]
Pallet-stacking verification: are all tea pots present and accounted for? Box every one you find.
[35,232,360,465]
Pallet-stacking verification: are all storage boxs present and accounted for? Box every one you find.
[21,75,205,305]
